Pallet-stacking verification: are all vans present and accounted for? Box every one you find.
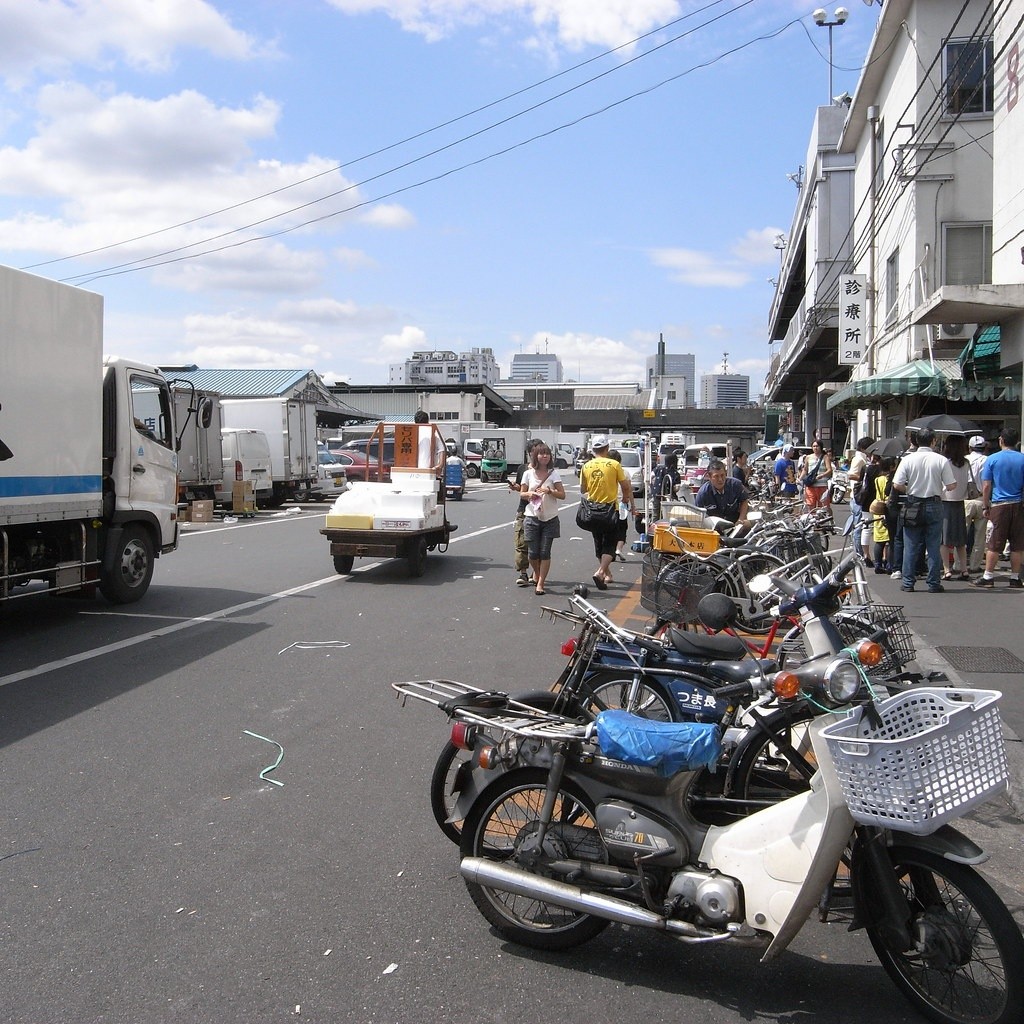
[658,443,685,466]
[343,438,395,462]
[215,427,275,509]
[293,444,349,502]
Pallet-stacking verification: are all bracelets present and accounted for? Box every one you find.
[738,520,743,523]
[547,486,552,495]
[982,506,989,510]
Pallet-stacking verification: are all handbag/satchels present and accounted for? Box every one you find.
[576,497,620,533]
[803,470,818,487]
[900,501,921,527]
[966,481,981,499]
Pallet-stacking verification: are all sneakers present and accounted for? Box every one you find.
[969,576,995,588]
[1009,577,1024,588]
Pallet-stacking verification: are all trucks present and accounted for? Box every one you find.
[0,265,212,613]
[470,428,646,473]
[346,423,493,478]
[133,388,224,510]
[220,398,322,505]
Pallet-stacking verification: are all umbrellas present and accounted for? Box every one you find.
[905,414,982,452]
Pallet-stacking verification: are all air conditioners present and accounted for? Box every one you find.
[937,324,978,340]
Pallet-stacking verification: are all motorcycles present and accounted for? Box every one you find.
[319,421,456,578]
[391,468,1024,1024]
[574,458,588,477]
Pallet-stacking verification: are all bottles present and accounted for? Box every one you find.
[641,533,645,542]
[619,498,626,520]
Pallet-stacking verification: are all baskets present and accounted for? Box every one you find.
[640,548,716,625]
[835,604,917,674]
[779,533,825,571]
[659,500,707,530]
[818,686,1010,836]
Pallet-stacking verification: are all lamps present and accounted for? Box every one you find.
[773,233,786,248]
[659,412,667,420]
[832,91,851,109]
[785,171,803,188]
[767,276,776,286]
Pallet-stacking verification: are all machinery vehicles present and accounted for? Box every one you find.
[480,437,508,484]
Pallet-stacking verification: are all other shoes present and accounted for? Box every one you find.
[886,569,892,575]
[604,578,613,582]
[592,575,607,591]
[532,579,538,586]
[890,571,901,579]
[864,557,874,567]
[874,568,886,574]
[928,585,944,593]
[516,573,529,585]
[535,587,544,595]
[615,550,626,561]
[900,585,914,592]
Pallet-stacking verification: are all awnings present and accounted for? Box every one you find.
[826,360,1022,416]
[956,320,1022,389]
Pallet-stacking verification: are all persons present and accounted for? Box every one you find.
[607,450,635,561]
[508,439,543,584]
[774,439,837,536]
[847,428,1024,593]
[445,448,468,494]
[520,444,565,596]
[134,417,148,430]
[414,411,445,477]
[580,435,630,590]
[574,445,595,460]
[695,460,750,527]
[732,449,752,487]
[649,453,681,524]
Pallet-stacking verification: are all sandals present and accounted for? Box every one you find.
[957,571,969,580]
[941,572,952,580]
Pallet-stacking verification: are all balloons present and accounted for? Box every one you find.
[866,437,909,457]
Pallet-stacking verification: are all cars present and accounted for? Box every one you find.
[329,449,391,481]
[613,448,643,496]
[747,445,813,466]
[683,443,728,465]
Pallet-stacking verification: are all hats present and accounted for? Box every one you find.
[969,435,986,447]
[592,435,608,449]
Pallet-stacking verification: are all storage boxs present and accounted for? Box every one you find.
[193,500,213,511]
[233,480,252,496]
[446,465,463,486]
[177,510,190,521]
[233,496,253,511]
[652,525,720,557]
[192,510,213,522]
[326,467,444,530]
